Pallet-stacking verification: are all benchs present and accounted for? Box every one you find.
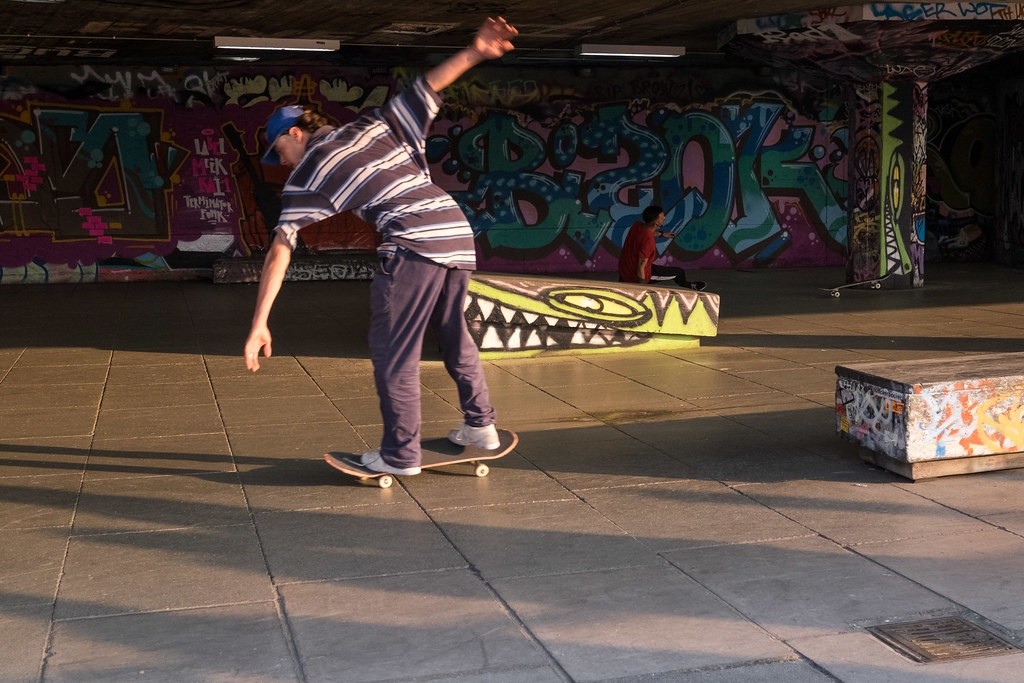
[462,272,720,359]
[835,351,1024,482]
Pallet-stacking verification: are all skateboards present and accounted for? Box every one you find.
[323,428,520,489]
[816,273,892,297]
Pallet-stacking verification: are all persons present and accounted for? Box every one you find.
[242,15,521,477]
[617,205,708,292]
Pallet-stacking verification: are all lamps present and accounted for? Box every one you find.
[579,43,686,58]
[213,36,340,52]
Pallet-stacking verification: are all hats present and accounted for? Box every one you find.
[259,105,305,167]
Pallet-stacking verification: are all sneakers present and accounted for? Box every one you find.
[361,450,421,476]
[447,424,500,450]
[689,281,707,291]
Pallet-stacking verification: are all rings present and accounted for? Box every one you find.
[249,352,253,358]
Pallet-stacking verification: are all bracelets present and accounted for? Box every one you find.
[660,230,664,238]
